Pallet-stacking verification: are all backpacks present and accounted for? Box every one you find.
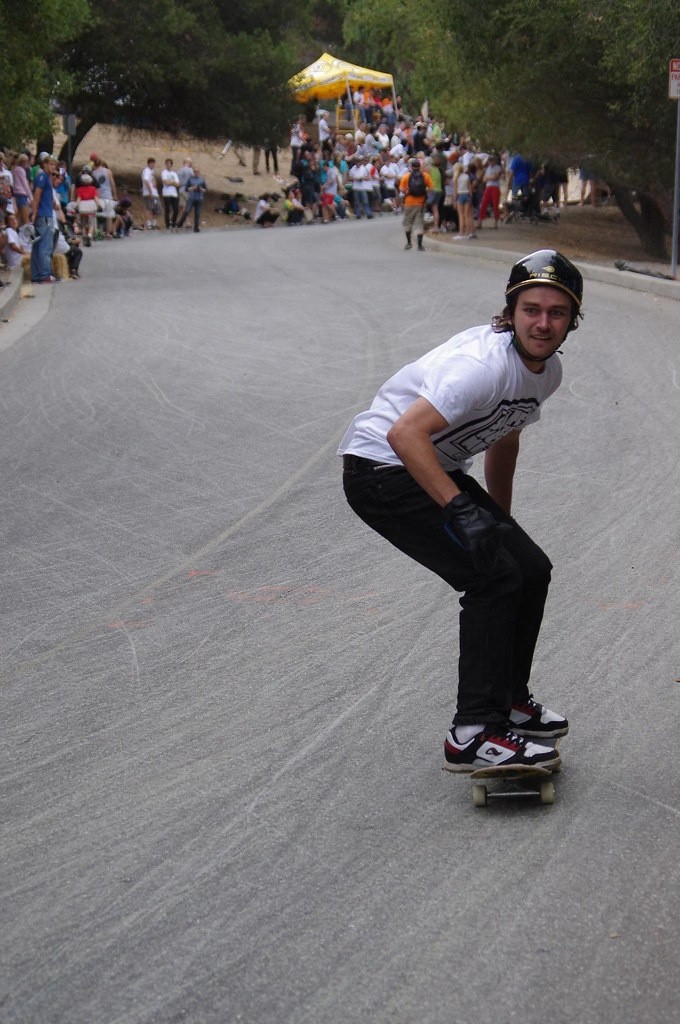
[406,169,426,199]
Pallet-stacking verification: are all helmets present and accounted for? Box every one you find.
[505,249,583,307]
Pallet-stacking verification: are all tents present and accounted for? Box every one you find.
[286,53,400,128]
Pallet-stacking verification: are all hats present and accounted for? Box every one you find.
[82,166,93,171]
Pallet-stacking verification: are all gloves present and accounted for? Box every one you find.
[443,491,516,570]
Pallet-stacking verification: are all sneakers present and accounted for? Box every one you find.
[443,724,561,773]
[506,687,569,739]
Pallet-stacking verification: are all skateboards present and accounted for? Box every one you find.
[470,733,561,807]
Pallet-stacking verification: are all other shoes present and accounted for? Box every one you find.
[367,214,373,218]
[42,275,62,282]
[405,242,412,248]
[356,216,361,218]
[31,278,42,283]
[308,219,317,224]
[71,270,80,279]
[452,234,464,240]
[83,235,92,247]
[418,246,425,250]
[254,171,262,175]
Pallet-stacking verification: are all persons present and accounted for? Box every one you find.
[400,160,433,249]
[161,159,180,229]
[177,158,207,232]
[224,85,614,241]
[336,248,584,774]
[141,159,162,229]
[0,143,133,287]
[31,157,57,283]
